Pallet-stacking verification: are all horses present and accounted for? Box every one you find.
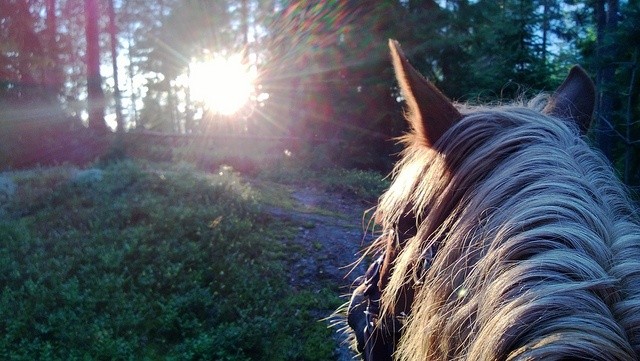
[317,38,640,361]
